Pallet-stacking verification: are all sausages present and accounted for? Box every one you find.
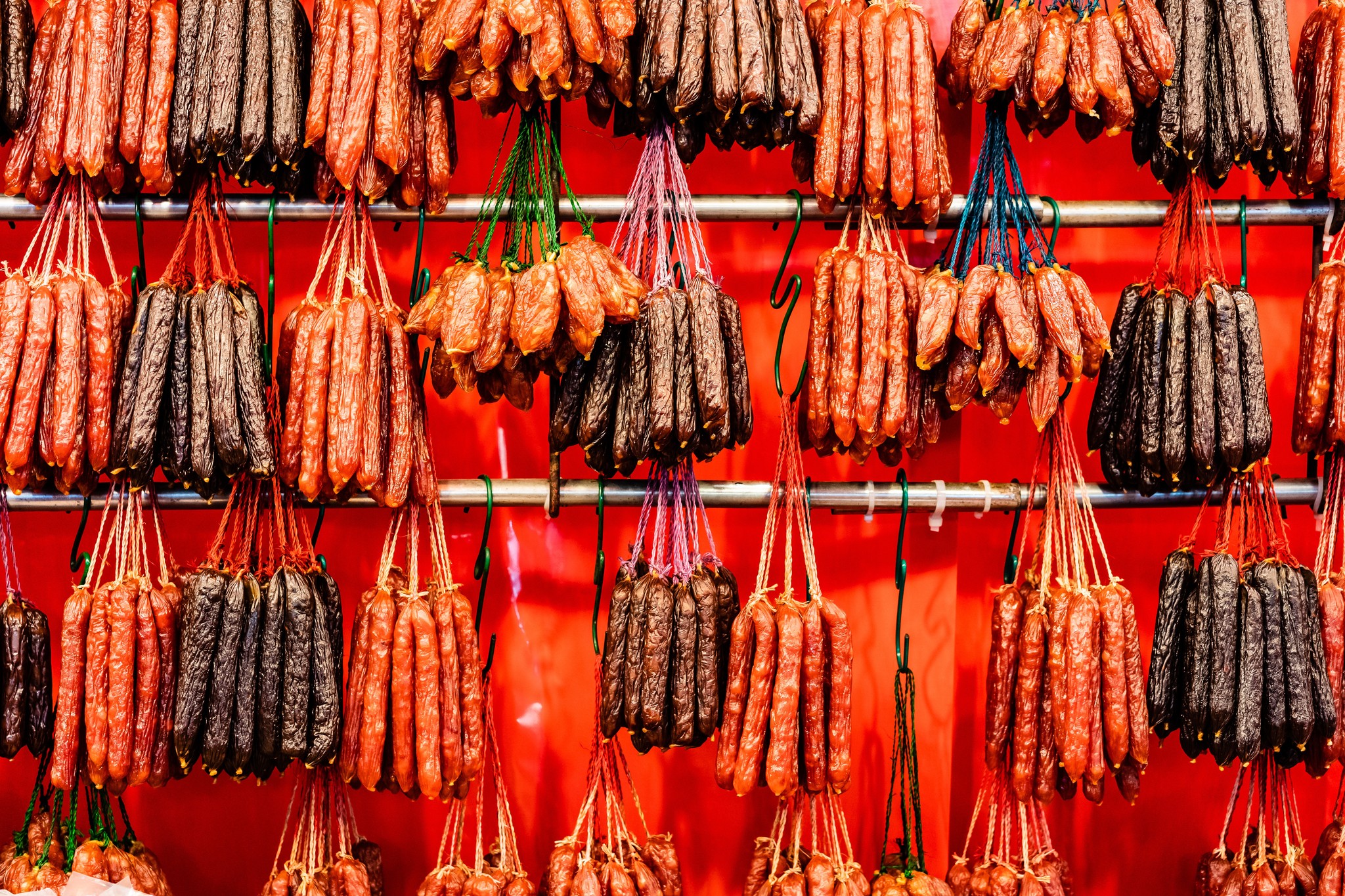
[0,1,1345,896]
